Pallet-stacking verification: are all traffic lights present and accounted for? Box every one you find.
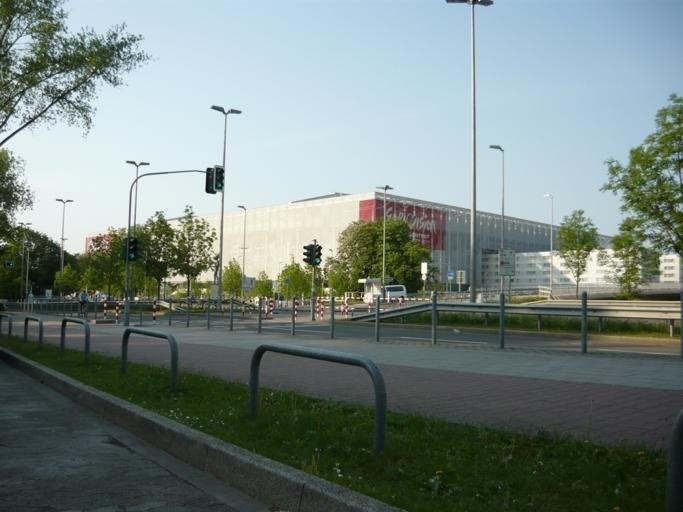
[213,164,225,192]
[302,244,322,267]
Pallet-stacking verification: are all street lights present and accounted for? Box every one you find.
[211,105,242,309]
[375,184,394,303]
[236,204,247,299]
[489,144,505,297]
[17,222,32,299]
[126,160,150,303]
[446,0,495,303]
[544,193,554,302]
[55,197,74,300]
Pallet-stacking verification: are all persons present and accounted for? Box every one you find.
[77,289,88,314]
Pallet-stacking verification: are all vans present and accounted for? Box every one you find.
[381,284,408,303]
